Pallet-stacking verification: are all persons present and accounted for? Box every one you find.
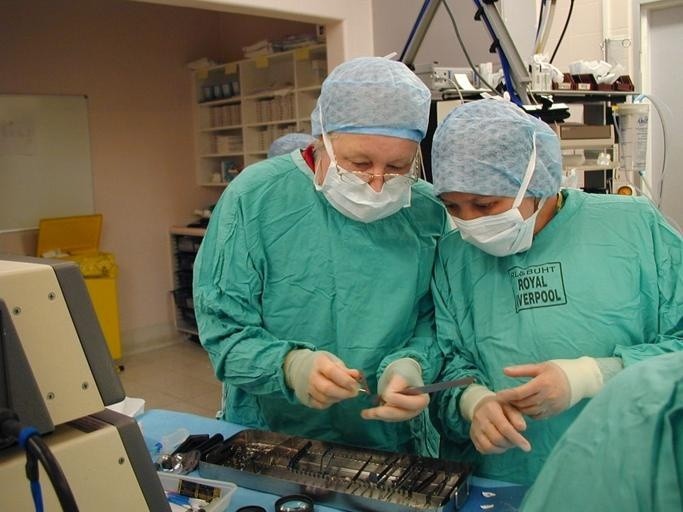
[193,54,449,463]
[429,100,682,488]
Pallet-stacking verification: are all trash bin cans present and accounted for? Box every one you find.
[36,213,123,375]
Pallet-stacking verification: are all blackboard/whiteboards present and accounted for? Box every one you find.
[0,93,95,233]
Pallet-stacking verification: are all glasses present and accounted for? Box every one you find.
[328,133,420,187]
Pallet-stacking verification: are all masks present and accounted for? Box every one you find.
[314,162,411,222]
[450,208,537,257]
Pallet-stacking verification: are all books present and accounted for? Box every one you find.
[198,83,316,181]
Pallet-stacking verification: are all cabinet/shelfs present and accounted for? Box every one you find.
[190,42,331,189]
[169,224,209,337]
